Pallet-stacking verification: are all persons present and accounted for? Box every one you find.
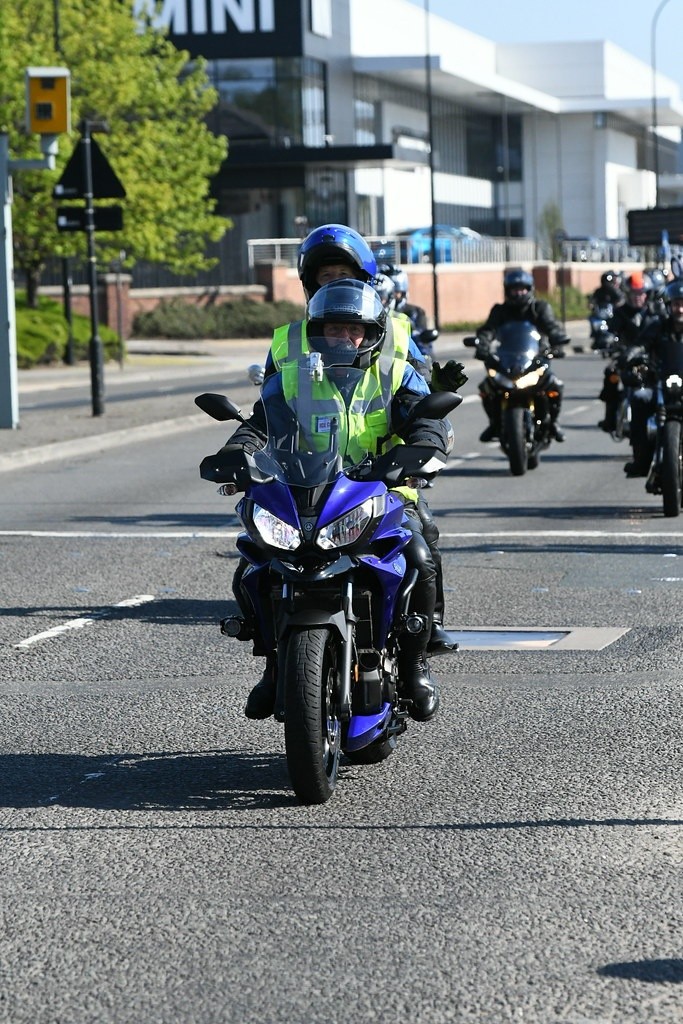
[216,278,449,721]
[478,271,571,442]
[260,225,471,657]
[587,266,683,477]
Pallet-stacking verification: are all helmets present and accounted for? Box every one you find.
[503,269,535,305]
[306,278,387,367]
[388,269,410,292]
[374,273,397,301]
[602,264,683,316]
[296,224,377,298]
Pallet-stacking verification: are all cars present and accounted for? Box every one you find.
[560,233,682,263]
[372,227,470,264]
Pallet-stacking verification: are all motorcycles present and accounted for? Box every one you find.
[192,364,463,802]
[621,352,682,518]
[463,332,569,476]
[588,294,620,358]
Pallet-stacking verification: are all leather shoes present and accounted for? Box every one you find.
[395,650,439,721]
[426,616,458,653]
[244,660,281,721]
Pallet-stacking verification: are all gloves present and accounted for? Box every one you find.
[430,361,469,393]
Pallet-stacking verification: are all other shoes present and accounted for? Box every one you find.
[480,422,501,440]
[551,420,565,441]
[598,386,648,477]
